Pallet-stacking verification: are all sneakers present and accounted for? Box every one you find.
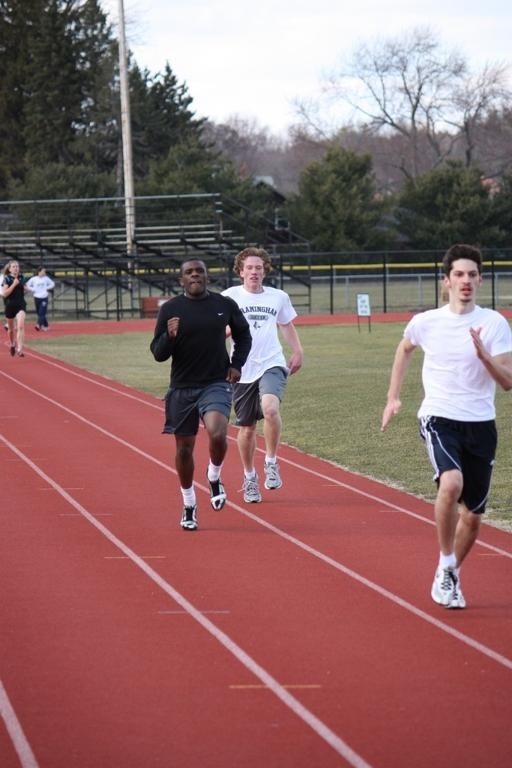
[431,561,466,608]
[10,341,16,355]
[206,465,226,510]
[264,460,282,490]
[180,504,199,530]
[243,473,261,503]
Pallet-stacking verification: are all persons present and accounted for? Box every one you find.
[150,257,253,531]
[380,244,511,610]
[24,268,56,332]
[214,247,303,504]
[0,259,31,358]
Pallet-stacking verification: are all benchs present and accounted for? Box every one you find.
[0,223,279,272]
